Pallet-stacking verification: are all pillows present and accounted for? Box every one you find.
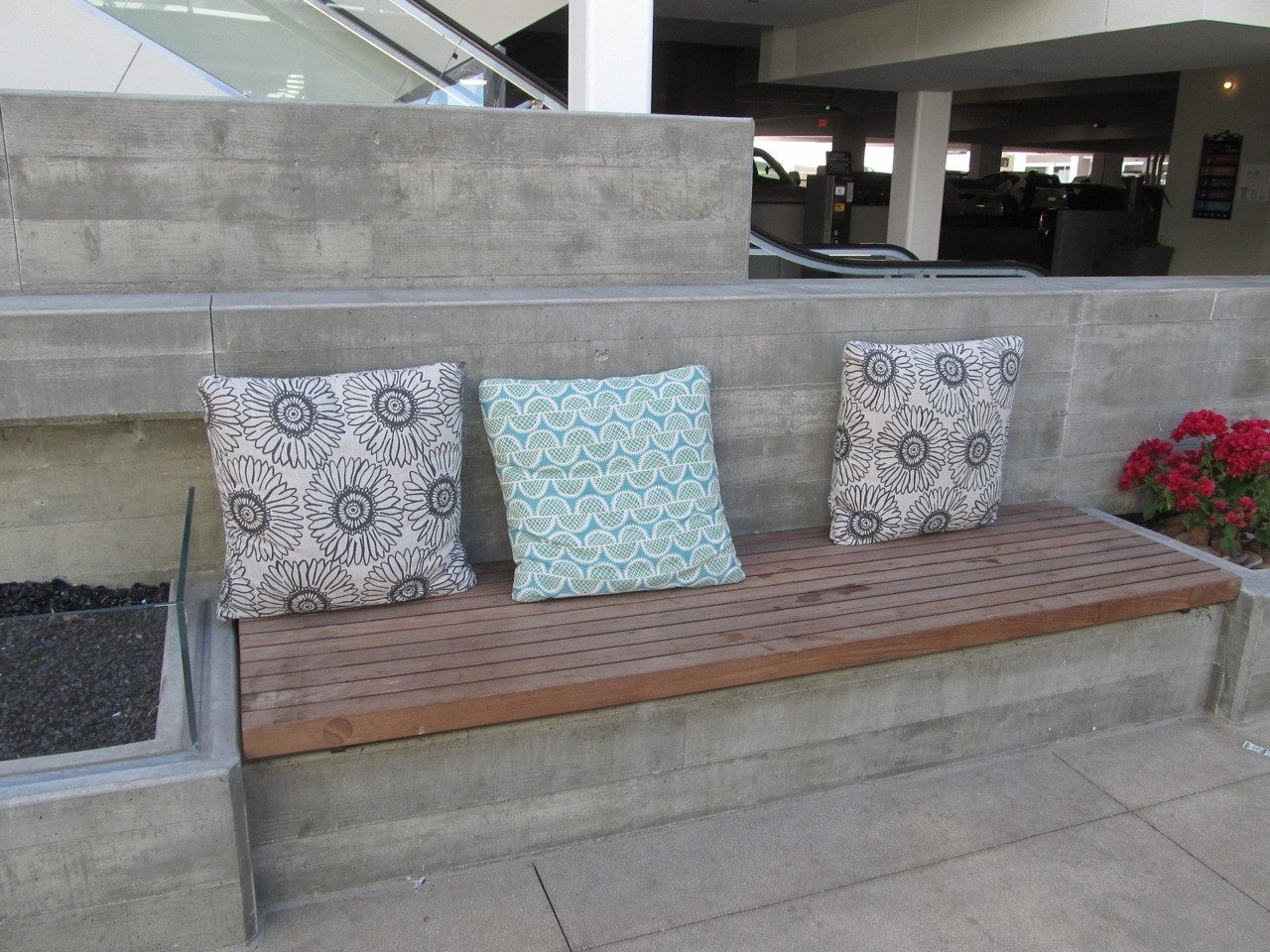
[474,365,746,602]
[827,336,1026,545]
[194,361,476,620]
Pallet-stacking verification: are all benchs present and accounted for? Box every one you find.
[242,500,1239,908]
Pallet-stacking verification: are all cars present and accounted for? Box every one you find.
[1036,184,1123,246]
[752,147,807,202]
[942,175,1018,227]
[854,185,872,204]
[866,172,892,206]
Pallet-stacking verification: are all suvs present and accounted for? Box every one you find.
[974,172,1062,210]
